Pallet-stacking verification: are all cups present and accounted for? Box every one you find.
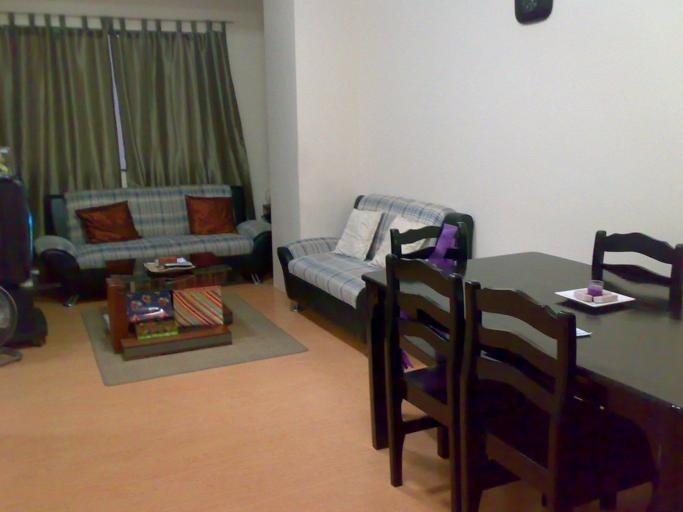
[587,279,604,300]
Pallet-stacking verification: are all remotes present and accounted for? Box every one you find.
[164,262,192,267]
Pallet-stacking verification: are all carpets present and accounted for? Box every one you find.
[79,289,309,387]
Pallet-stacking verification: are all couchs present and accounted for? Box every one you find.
[41,186,272,308]
[276,193,473,346]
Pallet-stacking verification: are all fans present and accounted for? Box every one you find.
[0,286,23,366]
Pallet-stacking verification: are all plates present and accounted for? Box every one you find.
[144,260,196,275]
[551,286,638,309]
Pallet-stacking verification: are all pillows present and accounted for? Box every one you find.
[186,193,238,236]
[331,207,384,262]
[74,201,143,244]
[374,214,429,269]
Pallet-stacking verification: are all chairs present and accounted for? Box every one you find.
[460,281,654,511]
[383,253,547,512]
[591,230,683,313]
[389,221,467,460]
